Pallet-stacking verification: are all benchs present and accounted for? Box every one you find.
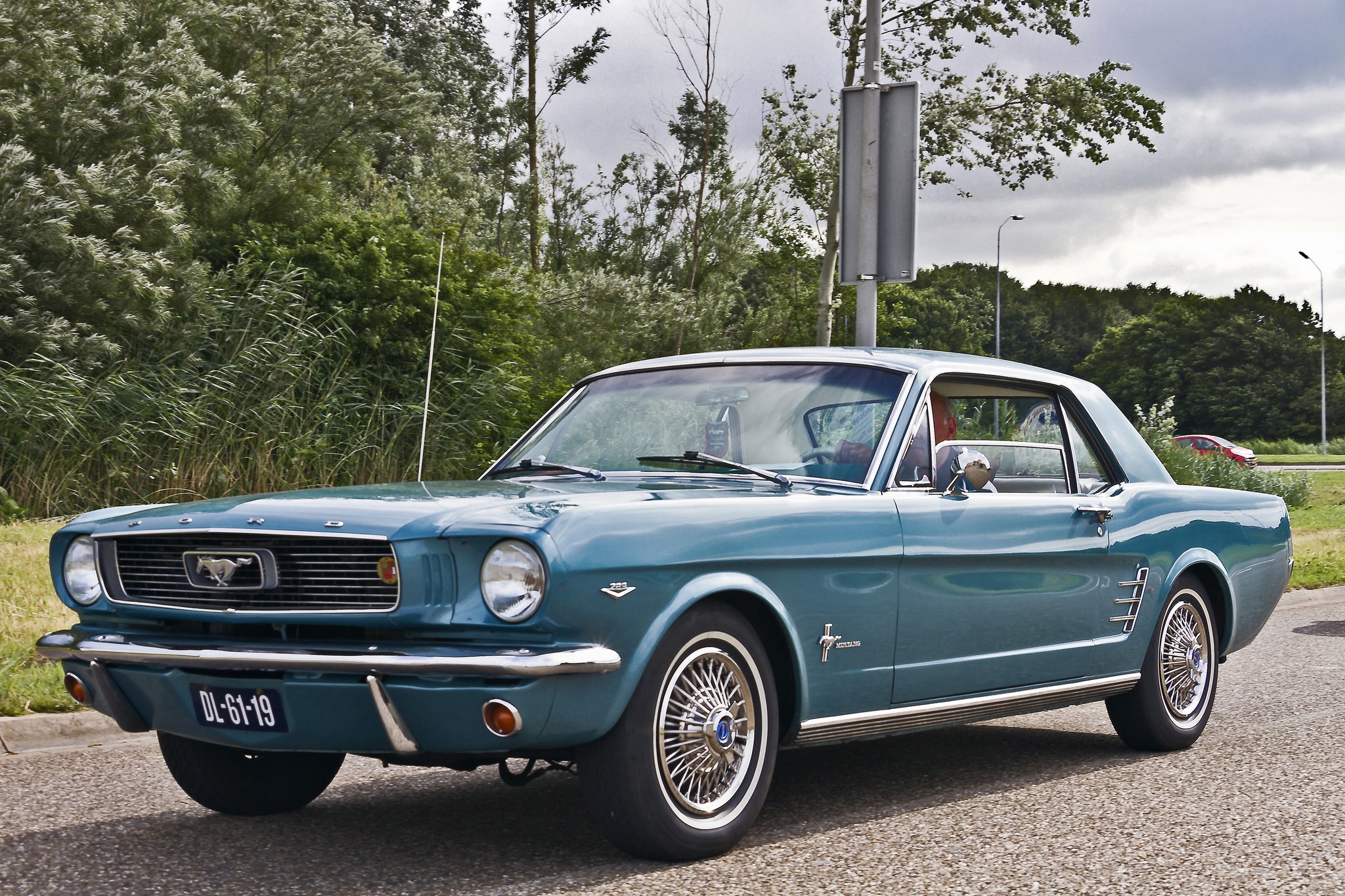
[986,471,1108,498]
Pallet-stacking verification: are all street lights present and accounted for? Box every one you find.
[1298,251,1327,455]
[995,215,1026,440]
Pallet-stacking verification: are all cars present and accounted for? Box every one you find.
[1172,435,1258,471]
[28,340,1290,866]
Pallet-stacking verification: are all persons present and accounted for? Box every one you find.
[834,391,995,492]
